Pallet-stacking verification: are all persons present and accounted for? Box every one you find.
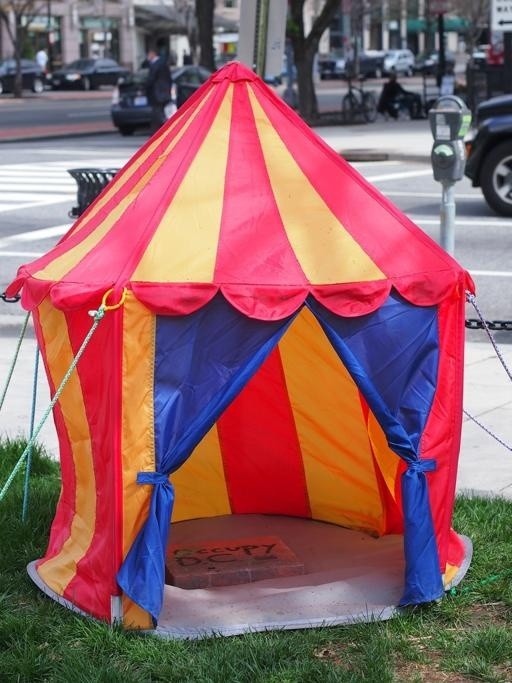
[144,47,172,136]
[387,74,425,119]
[36,47,49,90]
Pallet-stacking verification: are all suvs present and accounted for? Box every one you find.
[461,89,512,216]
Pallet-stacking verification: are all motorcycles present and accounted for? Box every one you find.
[378,64,422,121]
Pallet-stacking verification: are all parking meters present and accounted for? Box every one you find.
[428,95,472,256]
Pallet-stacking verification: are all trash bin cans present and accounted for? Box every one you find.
[67,169,121,220]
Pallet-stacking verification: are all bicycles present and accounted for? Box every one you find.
[340,62,376,125]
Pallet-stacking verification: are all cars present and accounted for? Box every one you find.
[211,47,456,89]
[46,57,130,90]
[0,57,48,93]
[466,44,492,67]
[110,65,213,137]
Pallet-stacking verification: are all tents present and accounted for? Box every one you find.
[4,62,475,642]
